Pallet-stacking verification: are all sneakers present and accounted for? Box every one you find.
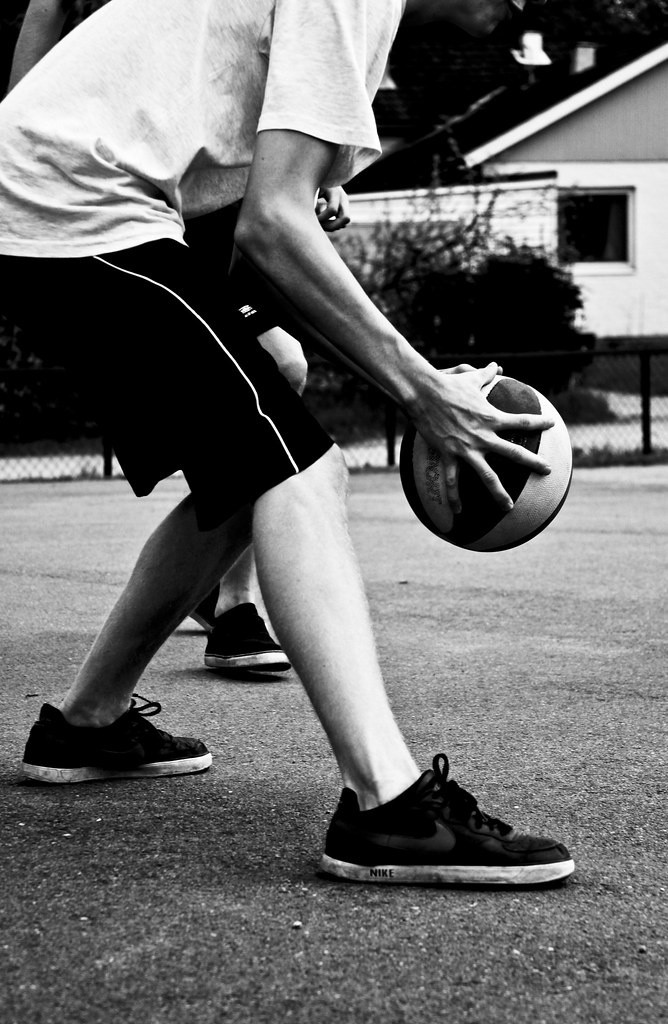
[188,580,292,672]
[321,753,575,884]
[21,694,212,783]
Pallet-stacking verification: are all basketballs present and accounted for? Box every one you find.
[397,369,577,555]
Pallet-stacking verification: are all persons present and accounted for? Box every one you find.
[3,0,580,885]
[5,1,353,675]
